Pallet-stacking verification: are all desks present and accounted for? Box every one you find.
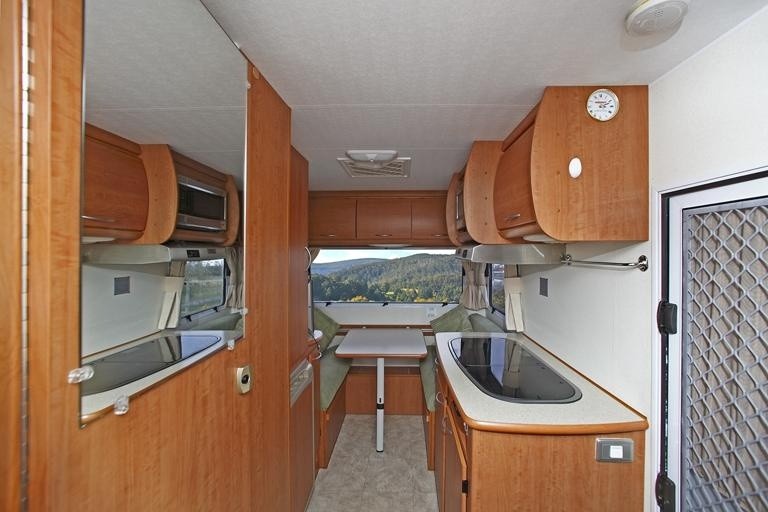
[333,326,429,452]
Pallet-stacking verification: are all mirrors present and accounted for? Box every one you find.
[78,0,248,430]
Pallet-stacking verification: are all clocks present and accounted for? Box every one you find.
[586,89,620,123]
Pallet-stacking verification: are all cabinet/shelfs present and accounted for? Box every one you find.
[290,145,310,374]
[308,190,456,247]
[433,356,650,512]
[463,85,649,241]
[445,139,514,248]
[345,365,427,415]
[85,119,178,245]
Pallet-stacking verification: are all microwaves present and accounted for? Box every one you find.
[176,174,227,231]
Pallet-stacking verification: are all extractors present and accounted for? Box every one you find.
[81,242,227,266]
[454,243,562,265]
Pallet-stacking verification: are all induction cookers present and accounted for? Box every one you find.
[80,334,221,396]
[448,338,582,404]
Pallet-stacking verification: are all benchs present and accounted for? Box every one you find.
[315,344,351,469]
[420,312,509,469]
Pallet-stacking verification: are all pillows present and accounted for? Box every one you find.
[430,305,471,334]
[313,304,339,351]
